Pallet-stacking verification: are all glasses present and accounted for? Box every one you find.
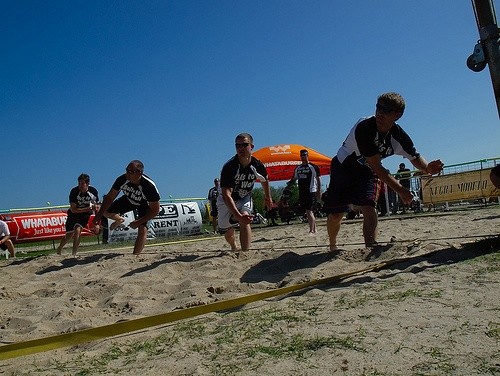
[234,142,252,148]
[300,153,308,156]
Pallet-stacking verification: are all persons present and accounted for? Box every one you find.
[217,133,273,252]
[92,160,160,255]
[208,178,220,234]
[0,220,15,257]
[377,170,390,217]
[395,163,410,214]
[57,173,100,255]
[285,150,322,234]
[324,92,445,252]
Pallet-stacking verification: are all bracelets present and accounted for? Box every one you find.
[265,198,272,201]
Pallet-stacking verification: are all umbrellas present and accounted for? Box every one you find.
[251,144,332,183]
